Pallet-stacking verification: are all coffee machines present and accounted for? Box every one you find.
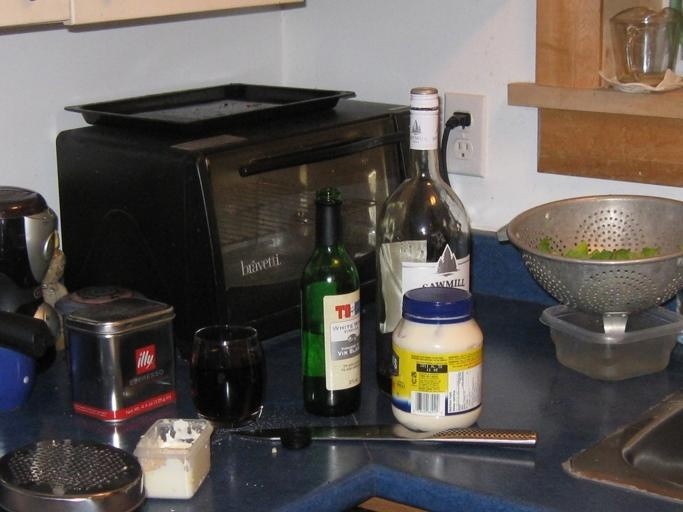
[0,185,67,368]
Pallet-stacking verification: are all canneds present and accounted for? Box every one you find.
[65,296,176,424]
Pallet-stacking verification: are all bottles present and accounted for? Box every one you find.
[385,286,484,431]
[376,86,472,393]
[299,185,362,419]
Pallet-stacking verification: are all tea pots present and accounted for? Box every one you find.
[607,7,681,85]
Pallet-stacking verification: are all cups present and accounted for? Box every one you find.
[187,323,266,428]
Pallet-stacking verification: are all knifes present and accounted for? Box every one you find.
[232,423,540,450]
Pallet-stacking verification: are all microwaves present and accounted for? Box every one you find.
[52,84,448,346]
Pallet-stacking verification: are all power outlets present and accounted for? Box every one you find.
[444,92,486,178]
